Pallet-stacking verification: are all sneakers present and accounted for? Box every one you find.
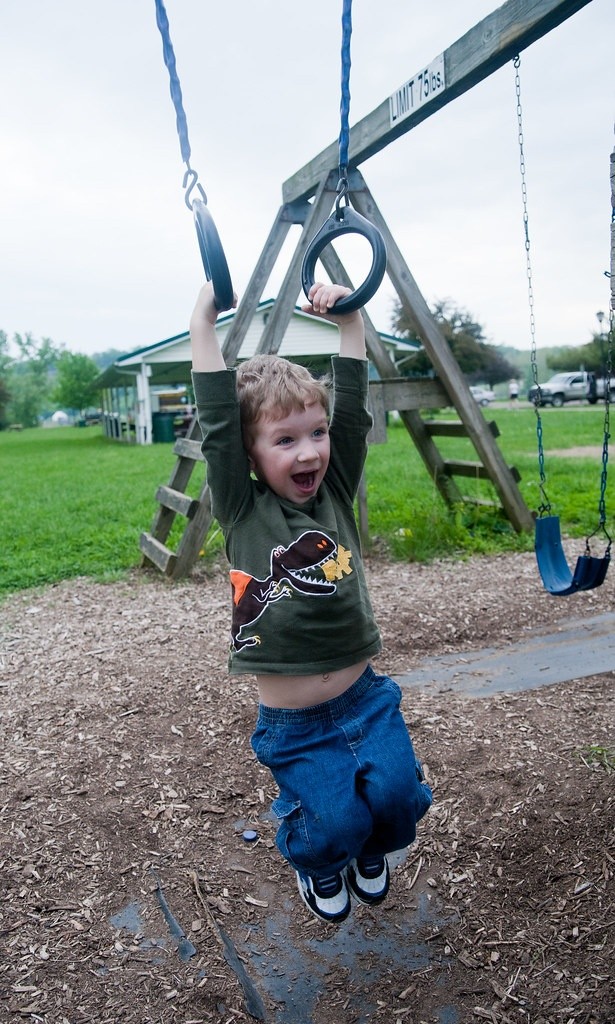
[343,853,390,907]
[297,869,351,924]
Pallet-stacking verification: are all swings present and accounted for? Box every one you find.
[507,57,615,597]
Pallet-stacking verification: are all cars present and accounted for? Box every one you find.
[469,387,495,407]
[527,373,615,408]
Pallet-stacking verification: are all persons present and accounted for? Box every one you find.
[508,379,519,410]
[189,279,435,924]
